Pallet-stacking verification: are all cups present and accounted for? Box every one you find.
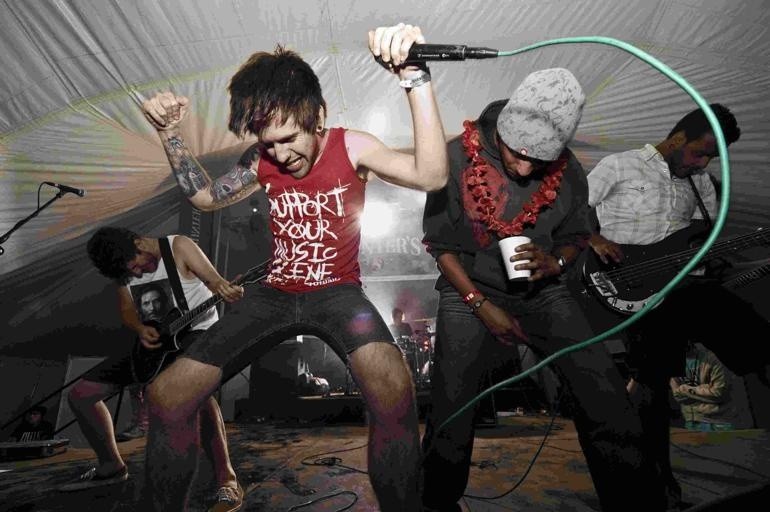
[497,235,533,284]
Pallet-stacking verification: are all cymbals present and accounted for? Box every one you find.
[410,318,434,324]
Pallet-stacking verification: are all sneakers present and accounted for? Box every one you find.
[207,479,245,511]
[61,465,129,491]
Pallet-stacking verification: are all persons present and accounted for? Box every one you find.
[420,67,682,512]
[7,406,54,457]
[388,308,418,379]
[669,344,736,431]
[144,23,448,512]
[59,227,245,512]
[133,282,168,322]
[586,104,770,473]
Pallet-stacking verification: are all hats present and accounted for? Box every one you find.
[496,68,586,164]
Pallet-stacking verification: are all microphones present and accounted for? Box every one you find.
[47,182,87,197]
[373,44,499,67]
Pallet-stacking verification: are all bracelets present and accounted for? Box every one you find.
[398,66,431,89]
[554,255,568,273]
[463,289,480,303]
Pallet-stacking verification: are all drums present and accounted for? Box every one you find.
[398,335,414,355]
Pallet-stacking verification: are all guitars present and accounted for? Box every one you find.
[568,222,770,318]
[131,257,274,387]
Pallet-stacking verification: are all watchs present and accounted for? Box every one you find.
[470,297,489,313]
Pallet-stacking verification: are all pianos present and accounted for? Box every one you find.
[0,439,70,461]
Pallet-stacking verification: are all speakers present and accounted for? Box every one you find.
[248,340,305,419]
